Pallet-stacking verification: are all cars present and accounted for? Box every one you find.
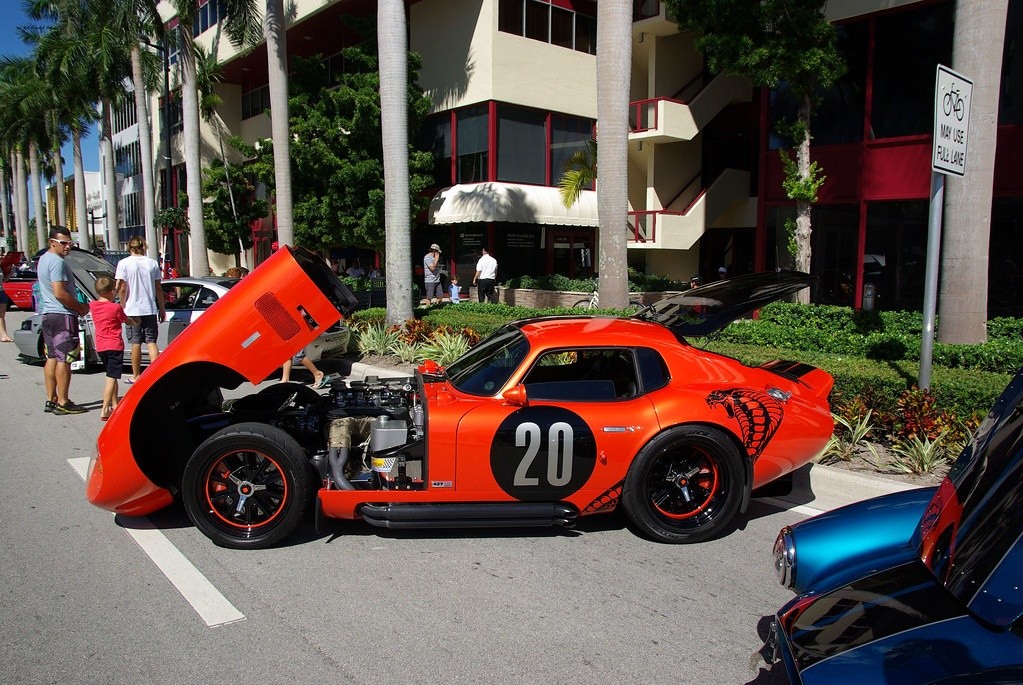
[14,276,243,374]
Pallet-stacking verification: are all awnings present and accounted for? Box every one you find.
[424,180,601,227]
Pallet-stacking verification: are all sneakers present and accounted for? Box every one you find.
[44,401,58,411]
[54,399,87,415]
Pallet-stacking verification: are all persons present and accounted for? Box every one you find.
[36,225,91,415]
[718,266,727,280]
[423,243,444,304]
[88,275,141,421]
[690,274,707,315]
[116,235,166,385]
[223,267,249,279]
[0,265,15,342]
[472,247,499,304]
[187,291,198,309]
[448,274,463,304]
[157,253,180,303]
[272,345,331,389]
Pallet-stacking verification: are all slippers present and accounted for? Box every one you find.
[101,417,109,421]
[124,378,135,384]
[314,376,330,388]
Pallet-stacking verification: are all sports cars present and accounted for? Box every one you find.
[756,355,1023,685]
[83,243,839,551]
[0,235,50,312]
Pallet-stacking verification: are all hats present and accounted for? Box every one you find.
[431,244,442,253]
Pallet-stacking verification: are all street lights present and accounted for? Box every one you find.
[136,36,176,271]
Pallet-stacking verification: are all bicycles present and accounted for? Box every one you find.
[569,278,646,318]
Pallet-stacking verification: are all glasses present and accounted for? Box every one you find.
[50,238,74,247]
